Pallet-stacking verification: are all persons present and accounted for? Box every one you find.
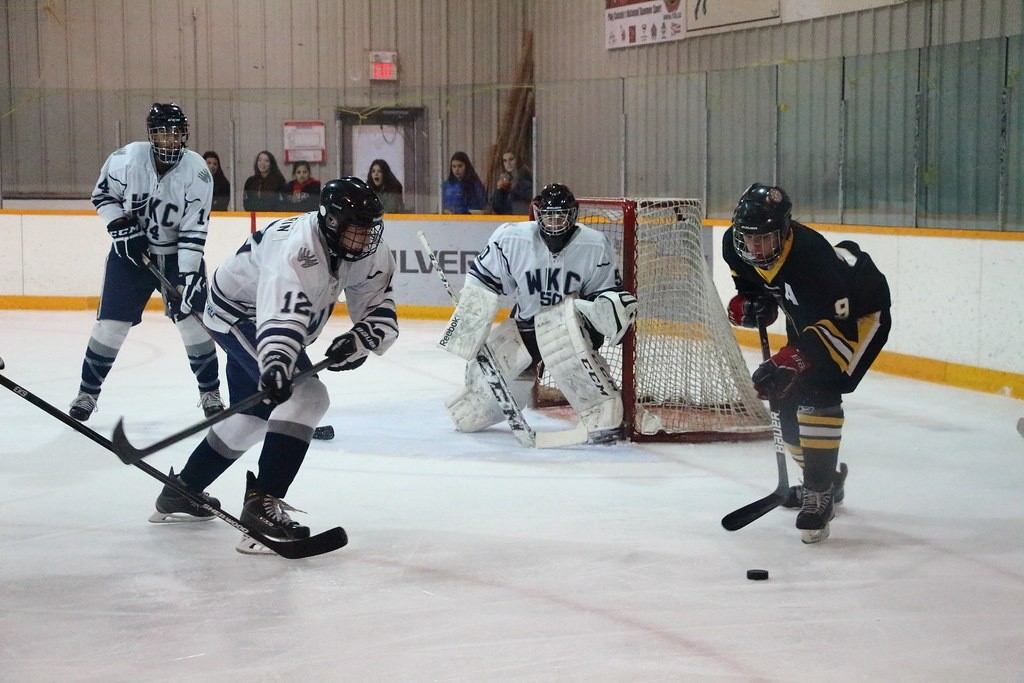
[436,183,637,449]
[365,159,406,213]
[202,151,230,212]
[69,103,225,425]
[439,151,490,214]
[243,151,285,211]
[722,182,891,548]
[489,147,533,215]
[279,161,322,212]
[146,176,399,554]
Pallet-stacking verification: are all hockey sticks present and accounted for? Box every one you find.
[111,355,336,465]
[416,230,590,448]
[720,314,789,532]
[0,373,349,561]
[141,252,335,442]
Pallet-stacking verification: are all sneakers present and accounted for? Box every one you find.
[70,382,101,431]
[796,483,835,544]
[782,462,847,510]
[148,466,220,523]
[236,470,310,555]
[198,380,225,418]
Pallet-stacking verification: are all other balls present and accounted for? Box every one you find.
[746,569,768,580]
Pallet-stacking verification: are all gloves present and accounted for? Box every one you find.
[170,275,207,324]
[728,295,765,329]
[752,346,811,401]
[261,351,293,405]
[106,217,151,271]
[326,321,385,372]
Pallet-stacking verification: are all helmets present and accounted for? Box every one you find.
[533,184,580,237]
[733,182,794,268]
[317,177,384,263]
[146,103,190,165]
